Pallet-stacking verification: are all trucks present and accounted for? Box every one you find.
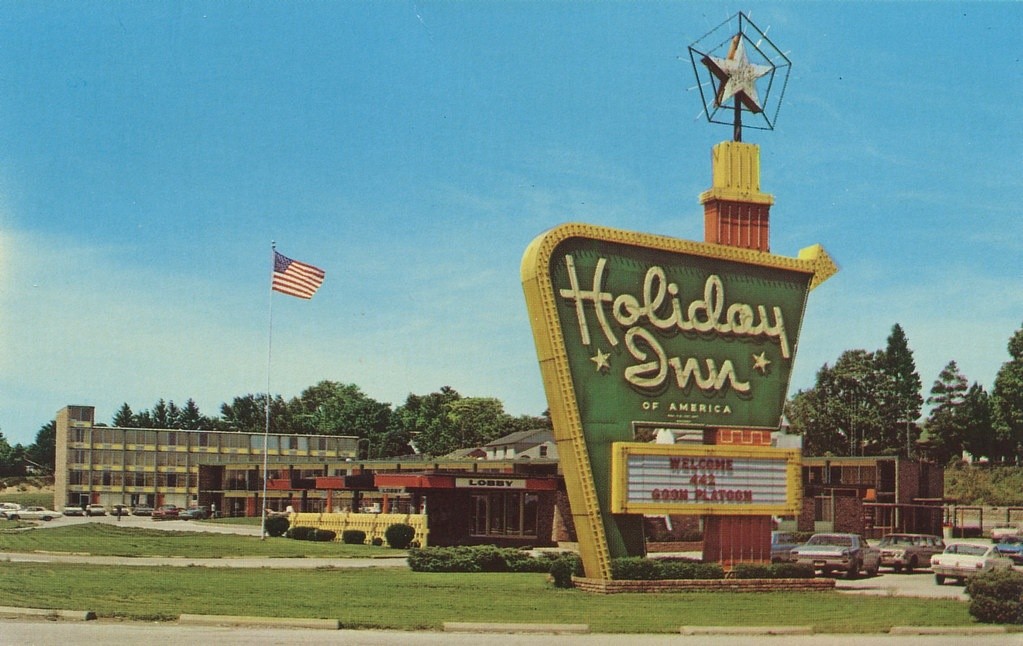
[363,502,393,513]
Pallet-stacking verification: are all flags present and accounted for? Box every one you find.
[273,250,326,299]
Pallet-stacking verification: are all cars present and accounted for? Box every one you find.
[178,506,211,521]
[943,521,982,538]
[930,542,1010,585]
[791,534,881,576]
[771,531,798,561]
[989,524,1021,543]
[26,466,41,475]
[86,503,106,516]
[110,504,129,516]
[8,507,62,521]
[132,504,187,520]
[0,502,21,517]
[63,503,83,515]
[875,533,946,572]
[995,536,1023,566]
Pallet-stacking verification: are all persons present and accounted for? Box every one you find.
[114,505,121,521]
[86,504,92,517]
[211,501,216,516]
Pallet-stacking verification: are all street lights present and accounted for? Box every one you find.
[824,451,834,484]
